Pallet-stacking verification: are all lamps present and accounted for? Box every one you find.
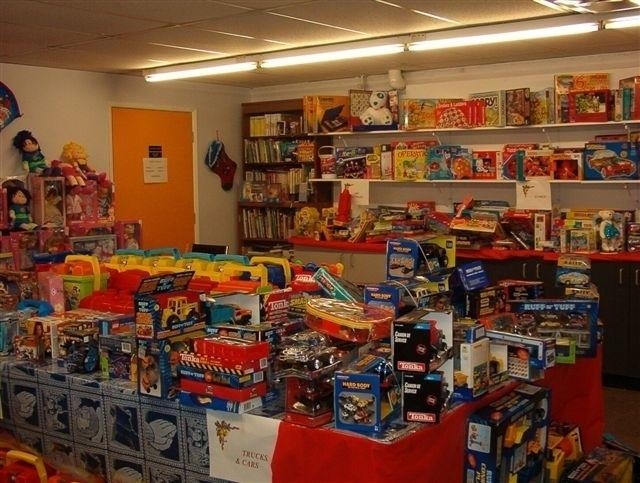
[144,60,259,83]
[605,15,639,30]
[406,23,601,55]
[259,42,407,69]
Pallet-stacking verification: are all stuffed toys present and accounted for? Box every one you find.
[599,210,619,251]
[124,223,138,250]
[20,224,72,272]
[360,91,393,125]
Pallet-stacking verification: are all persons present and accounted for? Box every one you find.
[33,322,45,338]
[8,187,41,231]
[51,140,113,221]
[13,130,61,177]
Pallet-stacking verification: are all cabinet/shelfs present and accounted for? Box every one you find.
[239,99,305,251]
[289,235,389,285]
[470,250,640,388]
[308,130,640,185]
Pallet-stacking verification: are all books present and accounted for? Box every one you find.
[241,113,314,239]
